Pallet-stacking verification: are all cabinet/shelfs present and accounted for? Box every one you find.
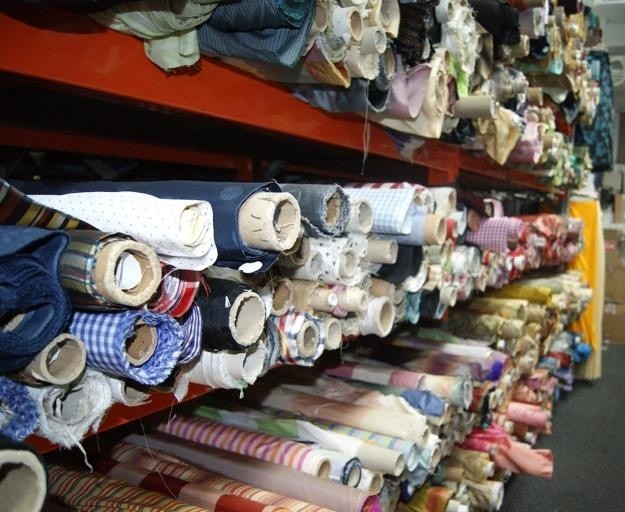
[1,6,567,463]
